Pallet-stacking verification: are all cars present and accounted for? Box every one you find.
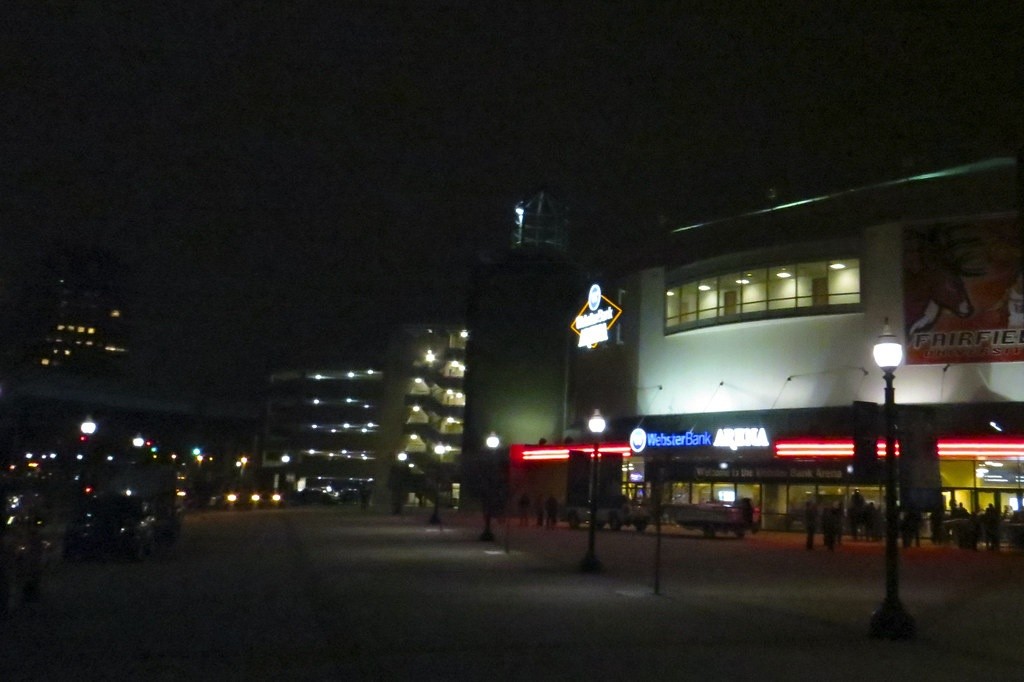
[63,462,184,565]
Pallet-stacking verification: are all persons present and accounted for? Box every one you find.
[804,499,1002,553]
[517,490,560,531]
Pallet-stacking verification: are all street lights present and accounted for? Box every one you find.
[576,407,608,575]
[871,315,929,643]
[478,428,501,542]
